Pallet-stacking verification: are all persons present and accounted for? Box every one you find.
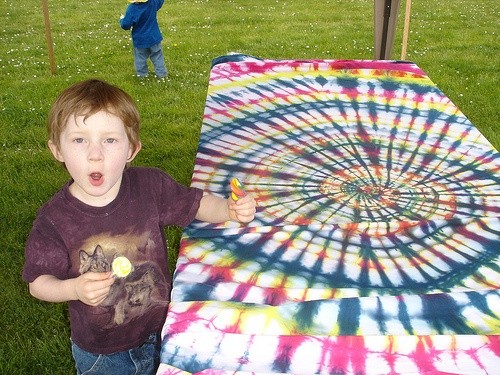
[120,0,167,78]
[22,78,257,375]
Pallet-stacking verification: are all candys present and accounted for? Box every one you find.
[231,177,246,201]
[108,256,132,279]
